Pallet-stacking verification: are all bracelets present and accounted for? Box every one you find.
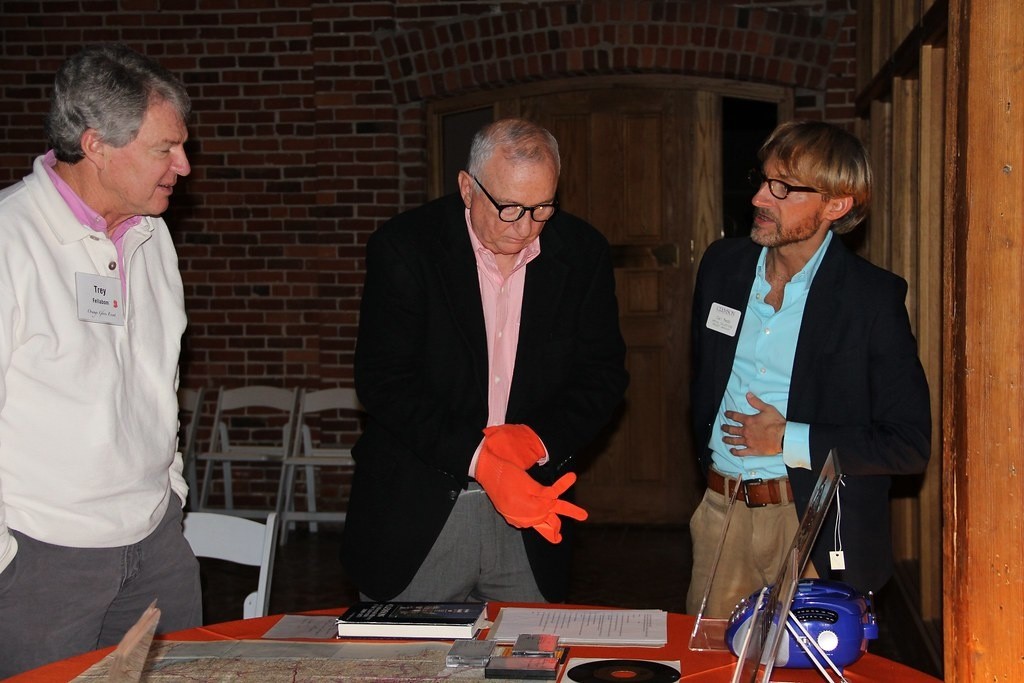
[781,435,784,450]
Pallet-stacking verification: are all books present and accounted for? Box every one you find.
[334,601,487,640]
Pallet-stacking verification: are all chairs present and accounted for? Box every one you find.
[182,511,279,620]
[280,388,369,547]
[196,383,300,530]
[176,383,205,511]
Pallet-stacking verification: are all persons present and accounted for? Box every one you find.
[685,117,932,616]
[0,41,203,682]
[333,117,630,604]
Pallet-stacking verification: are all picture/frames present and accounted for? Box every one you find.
[739,447,853,683]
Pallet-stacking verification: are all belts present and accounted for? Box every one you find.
[705,466,796,509]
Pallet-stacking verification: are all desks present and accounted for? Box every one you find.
[0,602,945,683]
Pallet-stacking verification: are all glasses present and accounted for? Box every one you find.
[748,166,823,200]
[471,173,559,223]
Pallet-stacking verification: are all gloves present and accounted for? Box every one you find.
[483,424,545,471]
[474,444,588,544]
